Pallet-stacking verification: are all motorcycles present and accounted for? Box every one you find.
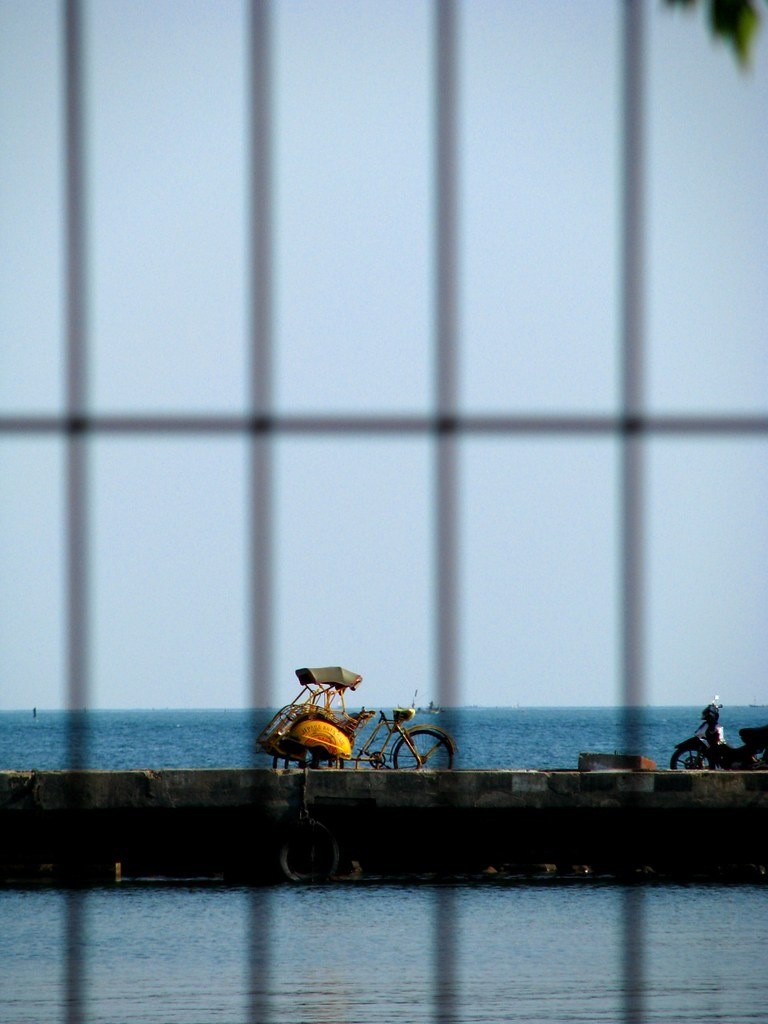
[670,694,768,770]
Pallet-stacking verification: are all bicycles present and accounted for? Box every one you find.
[272,705,454,770]
